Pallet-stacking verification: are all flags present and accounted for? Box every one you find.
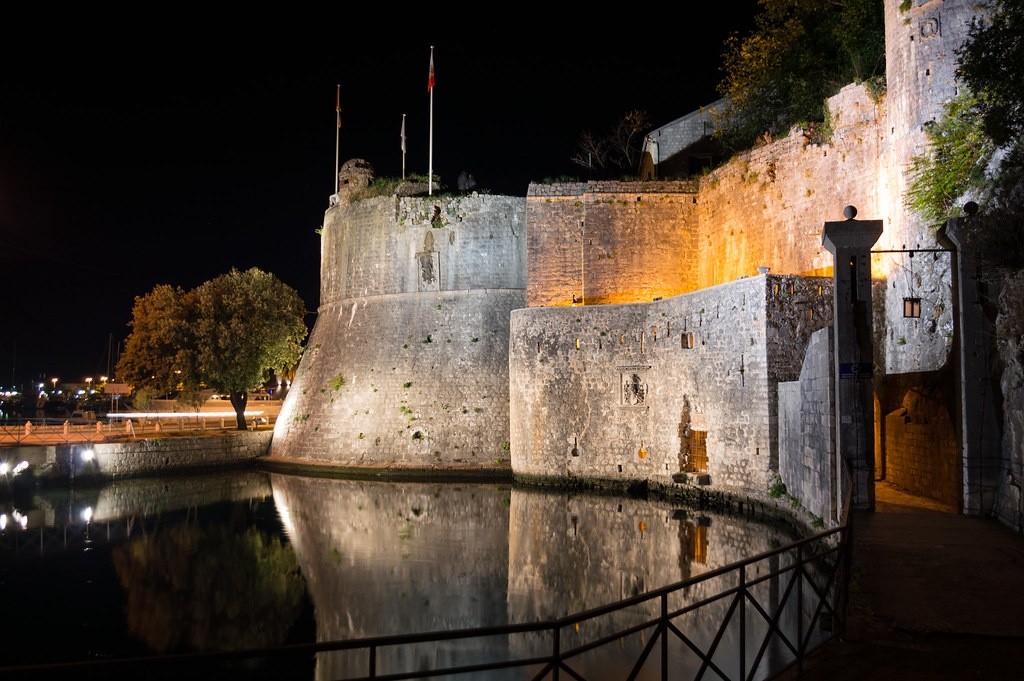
[336,84,342,128]
[428,46,435,92]
[401,114,407,154]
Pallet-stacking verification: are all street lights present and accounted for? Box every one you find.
[100,376,108,387]
[38,383,44,391]
[51,377,58,392]
[85,378,93,389]
